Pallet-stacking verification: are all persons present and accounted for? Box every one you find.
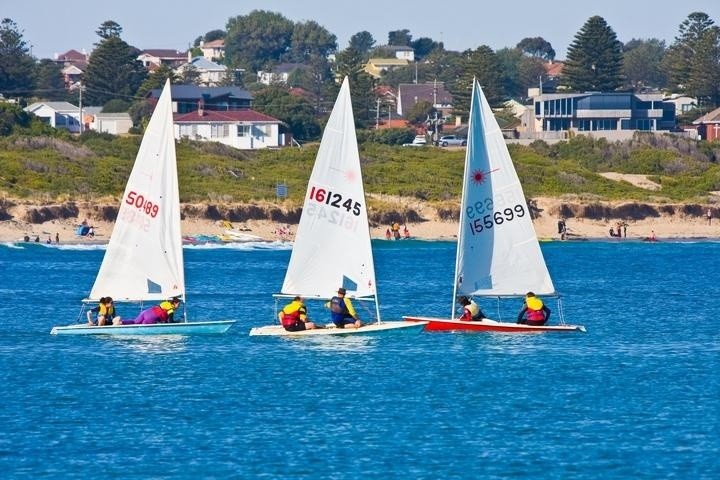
[707,209,712,226]
[609,221,629,237]
[77,219,95,239]
[458,295,486,322]
[87,297,116,326]
[118,297,180,325]
[650,230,656,239]
[278,296,316,331]
[386,221,411,241]
[325,289,362,328]
[272,223,293,236]
[24,232,60,245]
[560,222,566,240]
[518,291,551,325]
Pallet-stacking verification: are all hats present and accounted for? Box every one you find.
[339,288,346,294]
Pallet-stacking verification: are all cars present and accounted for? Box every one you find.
[411,134,427,144]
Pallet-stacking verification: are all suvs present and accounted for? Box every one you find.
[438,134,467,147]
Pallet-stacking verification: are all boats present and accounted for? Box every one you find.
[644,236,662,243]
[176,228,266,244]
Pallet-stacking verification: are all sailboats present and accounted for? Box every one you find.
[244,73,430,343]
[400,75,589,335]
[47,76,237,340]
